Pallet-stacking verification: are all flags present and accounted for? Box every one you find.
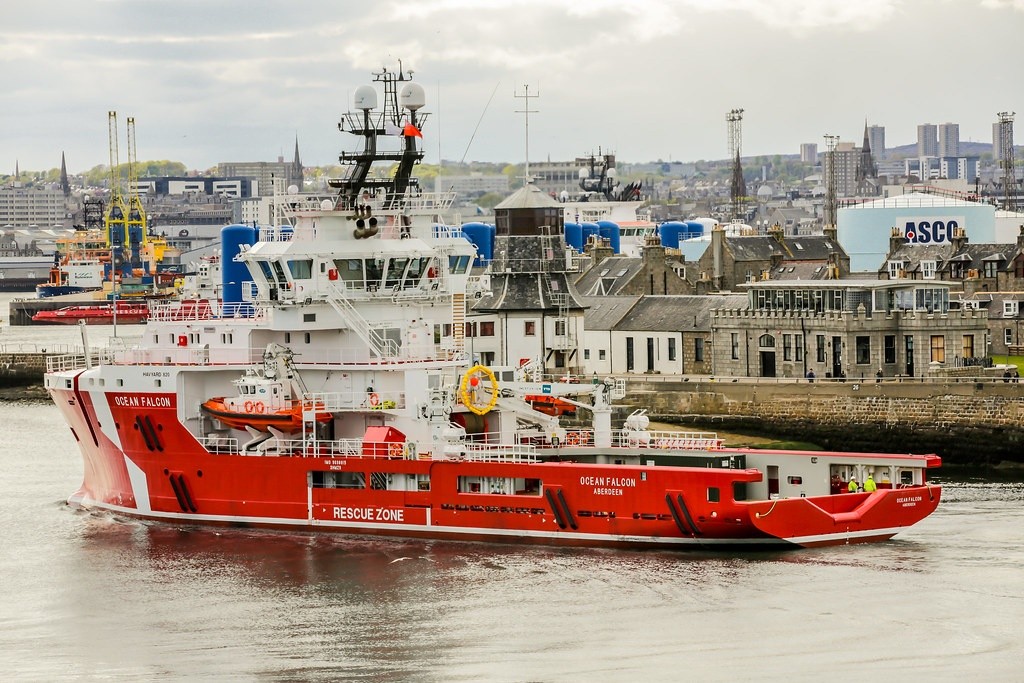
[386,122,402,134]
[404,121,423,139]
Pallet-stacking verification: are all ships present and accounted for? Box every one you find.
[33,58,943,560]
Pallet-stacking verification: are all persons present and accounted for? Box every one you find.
[876,368,883,382]
[831,475,841,494]
[864,476,876,492]
[848,476,858,493]
[807,368,815,383]
[1012,372,1019,384]
[1002,370,1012,383]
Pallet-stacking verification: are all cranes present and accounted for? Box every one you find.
[93,111,157,301]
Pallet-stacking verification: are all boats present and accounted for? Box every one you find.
[523,393,577,418]
[201,344,333,434]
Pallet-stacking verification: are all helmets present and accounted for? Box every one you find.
[868,476,872,478]
[851,476,855,480]
